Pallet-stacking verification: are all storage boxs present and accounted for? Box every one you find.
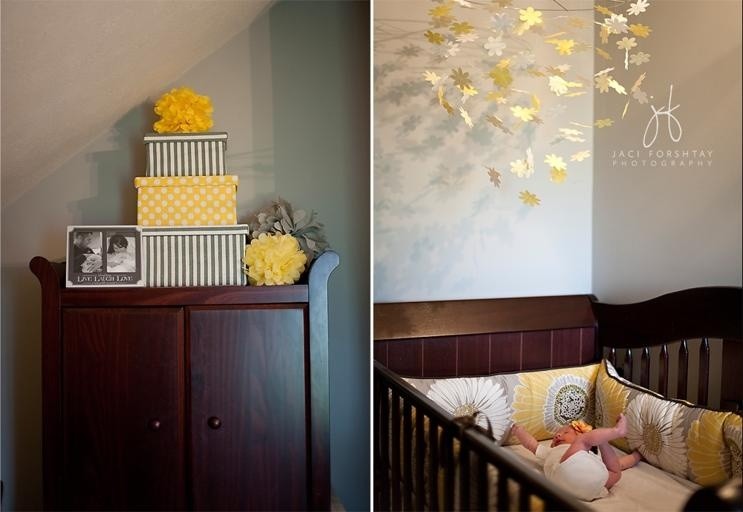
[133,131,250,285]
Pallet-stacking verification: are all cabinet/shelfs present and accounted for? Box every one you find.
[29,250,341,510]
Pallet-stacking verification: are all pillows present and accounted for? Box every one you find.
[378,356,741,488]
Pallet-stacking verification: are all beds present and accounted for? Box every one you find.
[370,284,742,511]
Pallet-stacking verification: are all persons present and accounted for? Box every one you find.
[512,412,641,502]
[107,235,136,272]
[73,232,103,273]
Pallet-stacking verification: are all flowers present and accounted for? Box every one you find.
[240,193,334,287]
[151,86,215,134]
